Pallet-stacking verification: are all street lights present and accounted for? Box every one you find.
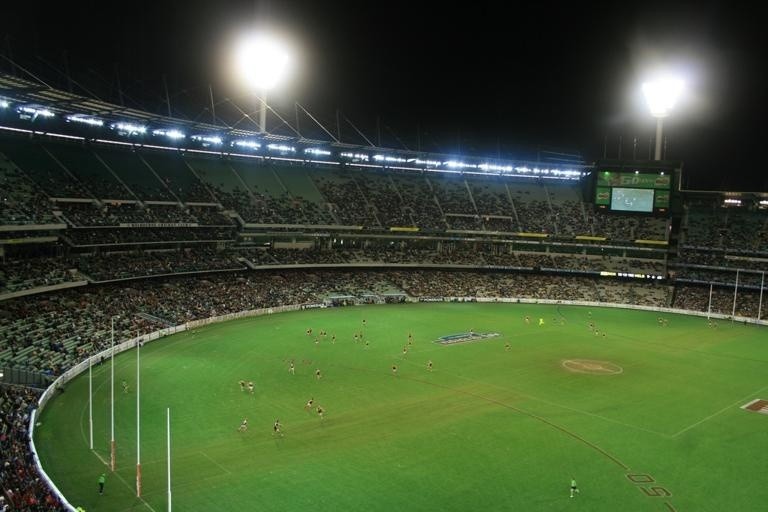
[644,75,676,160]
[240,40,287,132]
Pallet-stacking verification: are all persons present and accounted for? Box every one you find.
[87,170,767,376]
[247,381,257,396]
[238,379,247,392]
[315,369,321,382]
[236,416,248,432]
[569,477,580,498]
[120,379,130,393]
[270,418,285,437]
[97,471,107,496]
[1,128,87,512]
[317,403,326,421]
[305,398,316,409]
